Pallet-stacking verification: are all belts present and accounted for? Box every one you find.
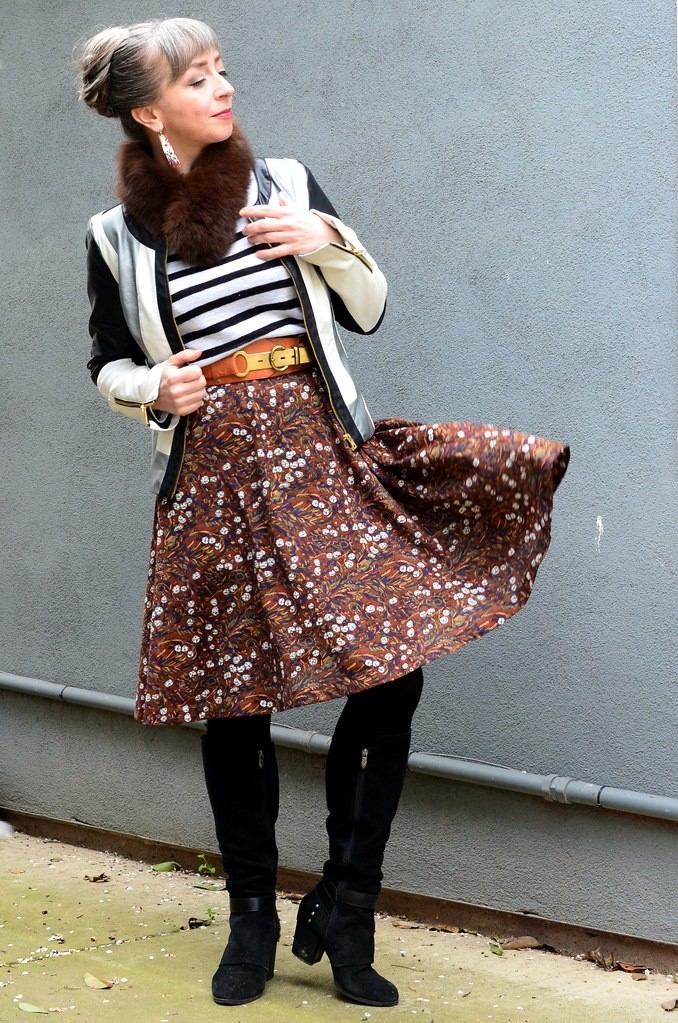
[201,336,316,387]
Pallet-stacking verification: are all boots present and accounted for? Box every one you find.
[200,732,281,1005]
[292,719,412,1008]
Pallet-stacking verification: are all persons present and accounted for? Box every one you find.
[74,17,570,1006]
